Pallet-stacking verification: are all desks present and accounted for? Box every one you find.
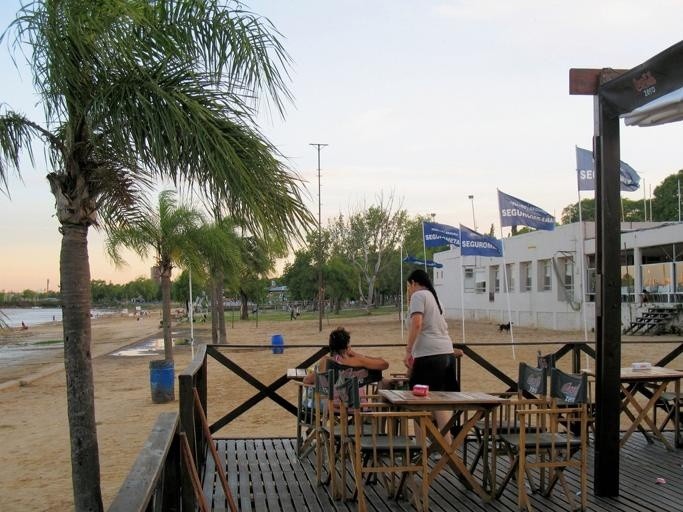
[578,367,681,454]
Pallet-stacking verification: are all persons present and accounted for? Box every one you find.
[303,327,389,427]
[403,269,458,464]
[289,305,296,320]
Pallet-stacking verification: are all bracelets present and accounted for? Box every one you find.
[406,346,412,353]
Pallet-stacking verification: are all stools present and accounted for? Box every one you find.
[652,389,682,449]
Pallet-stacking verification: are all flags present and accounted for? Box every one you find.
[575,146,641,191]
[402,256,443,268]
[422,221,459,248]
[497,190,556,231]
[459,224,503,258]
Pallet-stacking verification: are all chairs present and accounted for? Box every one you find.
[286,350,595,512]
